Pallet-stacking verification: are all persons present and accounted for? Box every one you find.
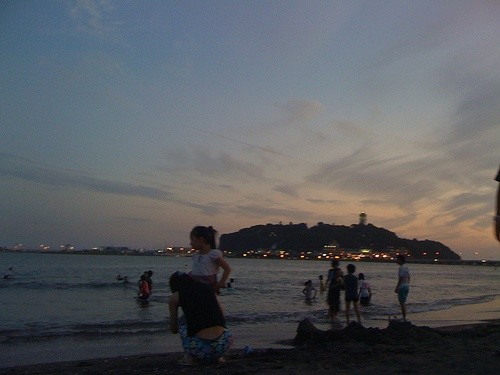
[136,270,153,305]
[326,258,360,323]
[168,270,234,365]
[358,273,372,303]
[189,225,232,295]
[302,280,318,299]
[319,274,323,287]
[394,255,410,322]
[227,278,234,288]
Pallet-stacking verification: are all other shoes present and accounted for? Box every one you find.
[217,359,226,364]
[177,359,193,366]
